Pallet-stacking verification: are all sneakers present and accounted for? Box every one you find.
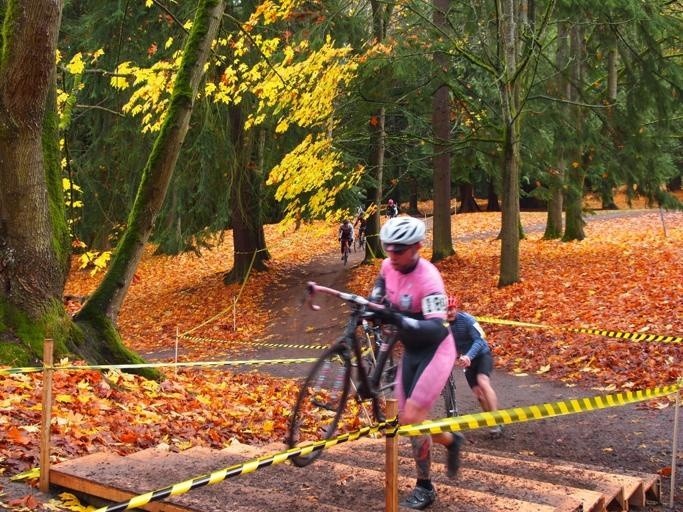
[446,430,466,478]
[487,424,509,434]
[400,484,437,509]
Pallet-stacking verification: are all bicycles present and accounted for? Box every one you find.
[341,228,366,267]
[440,352,466,417]
[387,213,394,219]
[289,281,400,467]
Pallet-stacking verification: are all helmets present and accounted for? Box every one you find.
[447,295,459,308]
[343,219,350,225]
[378,216,426,251]
[388,199,393,206]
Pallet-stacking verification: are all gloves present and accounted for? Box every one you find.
[357,297,383,319]
[458,355,472,367]
[375,309,418,330]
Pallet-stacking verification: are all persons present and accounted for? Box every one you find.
[354,212,369,249]
[338,219,355,260]
[363,215,458,511]
[445,294,506,435]
[385,199,398,218]
